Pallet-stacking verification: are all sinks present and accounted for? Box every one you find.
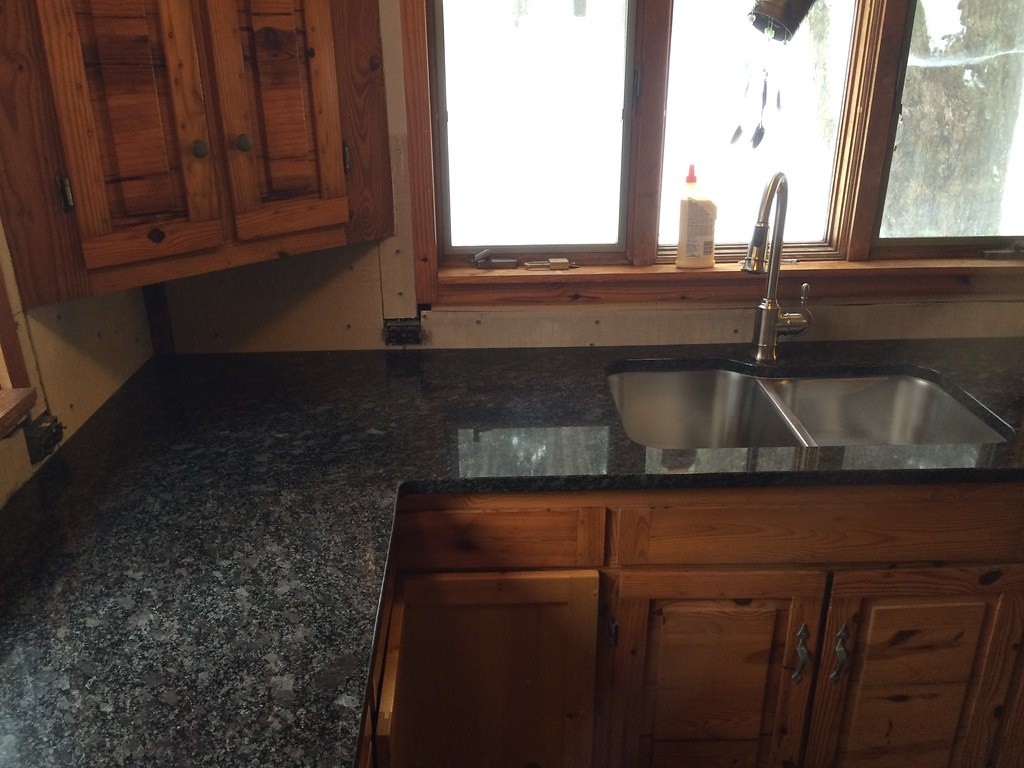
[603,357,819,450]
[755,362,1018,448]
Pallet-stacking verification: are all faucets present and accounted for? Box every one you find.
[739,170,790,363]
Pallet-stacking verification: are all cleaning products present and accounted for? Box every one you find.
[674,162,719,269]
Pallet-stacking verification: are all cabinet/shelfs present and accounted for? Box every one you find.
[356,479,1023,768]
[0,0,399,307]
